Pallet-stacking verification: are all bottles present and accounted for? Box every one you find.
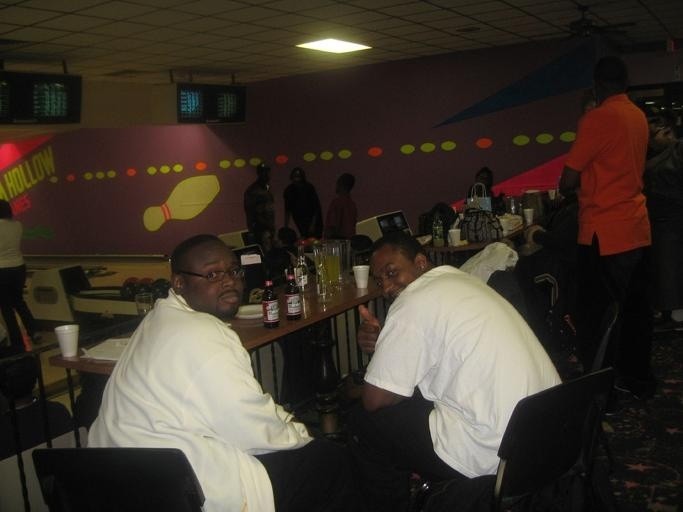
[294,245,308,295]
[285,274,301,319]
[432,212,444,248]
[262,279,279,328]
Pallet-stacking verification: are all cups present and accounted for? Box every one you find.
[448,229,461,246]
[330,239,351,287]
[55,325,79,358]
[522,207,535,227]
[353,265,370,289]
[547,188,558,200]
[312,249,327,304]
[135,293,154,321]
[322,246,339,297]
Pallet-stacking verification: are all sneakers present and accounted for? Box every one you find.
[605,400,625,418]
[617,379,654,400]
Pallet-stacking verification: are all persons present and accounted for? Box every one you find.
[244,163,274,247]
[283,168,322,238]
[340,229,562,480]
[260,226,317,281]
[323,173,357,239]
[0,199,43,355]
[88,234,343,511]
[560,57,653,416]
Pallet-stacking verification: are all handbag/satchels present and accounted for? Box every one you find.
[466,183,491,210]
[460,207,505,242]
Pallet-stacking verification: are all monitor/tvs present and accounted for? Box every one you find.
[16,73,81,124]
[206,85,246,123]
[1,72,20,123]
[176,81,211,124]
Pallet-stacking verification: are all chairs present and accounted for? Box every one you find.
[29,448,205,512]
[0,353,72,512]
[560,281,623,376]
[415,364,614,512]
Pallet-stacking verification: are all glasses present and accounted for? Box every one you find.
[180,266,246,282]
